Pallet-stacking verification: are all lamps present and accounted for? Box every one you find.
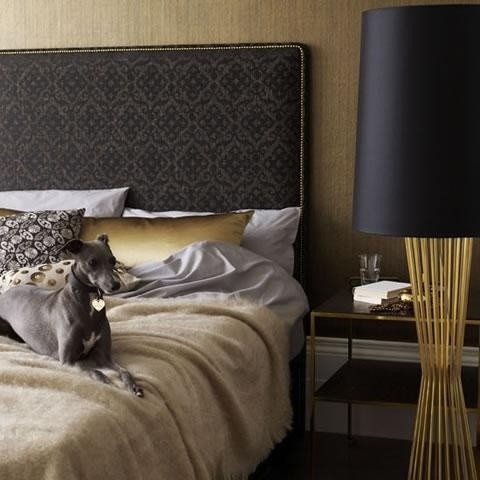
[351,4,479,480]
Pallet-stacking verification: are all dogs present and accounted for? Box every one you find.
[0,234,144,397]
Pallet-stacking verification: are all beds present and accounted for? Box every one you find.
[0,40,313,480]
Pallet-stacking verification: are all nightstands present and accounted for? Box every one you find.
[305,283,480,449]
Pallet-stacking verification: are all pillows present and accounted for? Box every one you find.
[80,211,255,268]
[119,204,304,278]
[3,207,87,279]
[3,187,130,217]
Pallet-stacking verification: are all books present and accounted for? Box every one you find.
[351,279,445,307]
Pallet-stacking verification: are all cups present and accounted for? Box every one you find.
[359,253,383,284]
